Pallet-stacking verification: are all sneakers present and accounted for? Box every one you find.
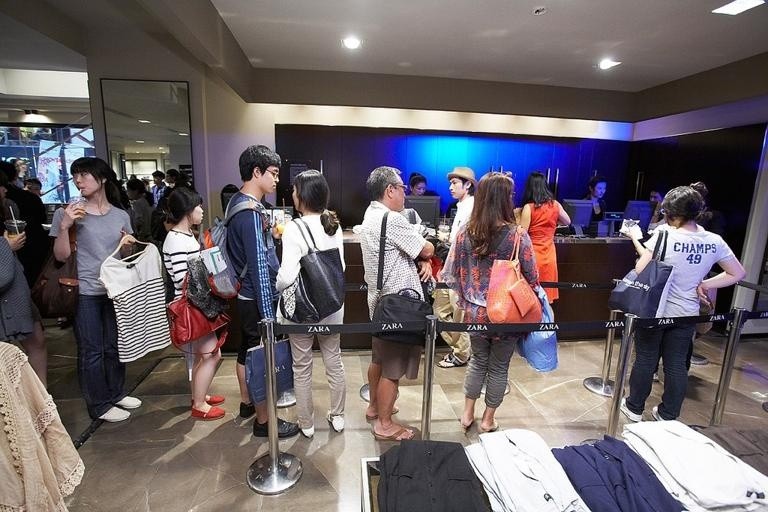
[620,397,642,421]
[652,406,666,421]
[192,395,225,420]
[437,357,467,367]
[96,395,142,421]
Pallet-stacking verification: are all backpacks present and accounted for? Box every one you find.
[198,191,270,299]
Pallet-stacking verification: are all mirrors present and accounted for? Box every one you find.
[99,78,195,204]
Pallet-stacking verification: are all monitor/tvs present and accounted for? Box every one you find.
[562,199,593,237]
[404,195,440,227]
[619,200,658,232]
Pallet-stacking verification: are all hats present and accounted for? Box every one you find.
[448,167,478,188]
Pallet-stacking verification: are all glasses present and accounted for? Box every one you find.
[661,208,667,215]
[397,184,407,190]
[265,168,280,178]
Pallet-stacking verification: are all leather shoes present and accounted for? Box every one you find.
[302,425,314,438]
[327,409,344,432]
[238,403,299,437]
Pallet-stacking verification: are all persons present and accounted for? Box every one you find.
[620,182,709,382]
[1,158,54,394]
[620,186,747,422]
[358,166,435,441]
[439,172,540,434]
[521,172,571,321]
[432,167,479,367]
[408,172,434,196]
[582,175,609,222]
[101,142,346,436]
[650,187,667,202]
[398,208,422,225]
[48,159,143,423]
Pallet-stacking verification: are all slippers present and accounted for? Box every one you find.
[461,417,473,433]
[366,406,398,419]
[374,425,415,440]
[478,418,498,433]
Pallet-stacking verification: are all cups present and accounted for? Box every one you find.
[284,214,293,224]
[272,210,285,235]
[4,220,27,238]
[68,196,89,219]
[438,225,449,233]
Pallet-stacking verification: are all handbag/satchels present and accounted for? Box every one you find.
[608,231,673,318]
[245,336,294,402]
[486,224,542,325]
[165,273,231,354]
[280,218,346,322]
[370,288,434,345]
[32,205,78,318]
